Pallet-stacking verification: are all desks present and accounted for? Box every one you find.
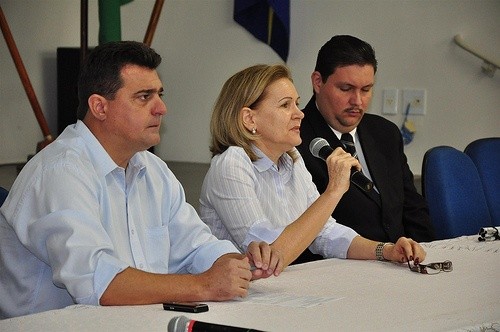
[0,235,500,332]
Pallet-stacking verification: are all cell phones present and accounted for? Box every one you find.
[162,303,208,313]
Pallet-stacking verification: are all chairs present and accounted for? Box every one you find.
[464,137,500,229]
[421,146,492,239]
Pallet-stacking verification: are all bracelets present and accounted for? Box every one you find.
[375,242,394,263]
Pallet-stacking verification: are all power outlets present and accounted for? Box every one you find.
[401,89,427,114]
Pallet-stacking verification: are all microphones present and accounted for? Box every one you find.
[309,137,374,192]
[167,315,266,332]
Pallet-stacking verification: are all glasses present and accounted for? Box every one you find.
[404,254,453,275]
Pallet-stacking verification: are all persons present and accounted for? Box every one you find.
[283,34,435,266]
[196,64,425,271]
[0,40,285,320]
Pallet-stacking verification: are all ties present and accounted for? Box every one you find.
[341,133,363,173]
